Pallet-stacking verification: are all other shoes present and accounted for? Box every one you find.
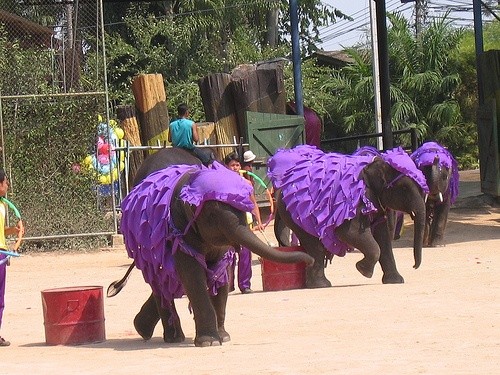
[242,288,253,294]
[0,336,10,346]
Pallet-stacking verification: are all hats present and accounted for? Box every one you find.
[243,150,256,162]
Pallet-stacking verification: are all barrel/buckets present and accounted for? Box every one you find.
[260,246,307,292]
[41,286,106,346]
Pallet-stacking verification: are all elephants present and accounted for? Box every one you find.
[106,142,458,346]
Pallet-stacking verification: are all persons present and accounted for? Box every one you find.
[225,152,266,295]
[167,103,212,168]
[242,150,256,187]
[0,168,25,348]
